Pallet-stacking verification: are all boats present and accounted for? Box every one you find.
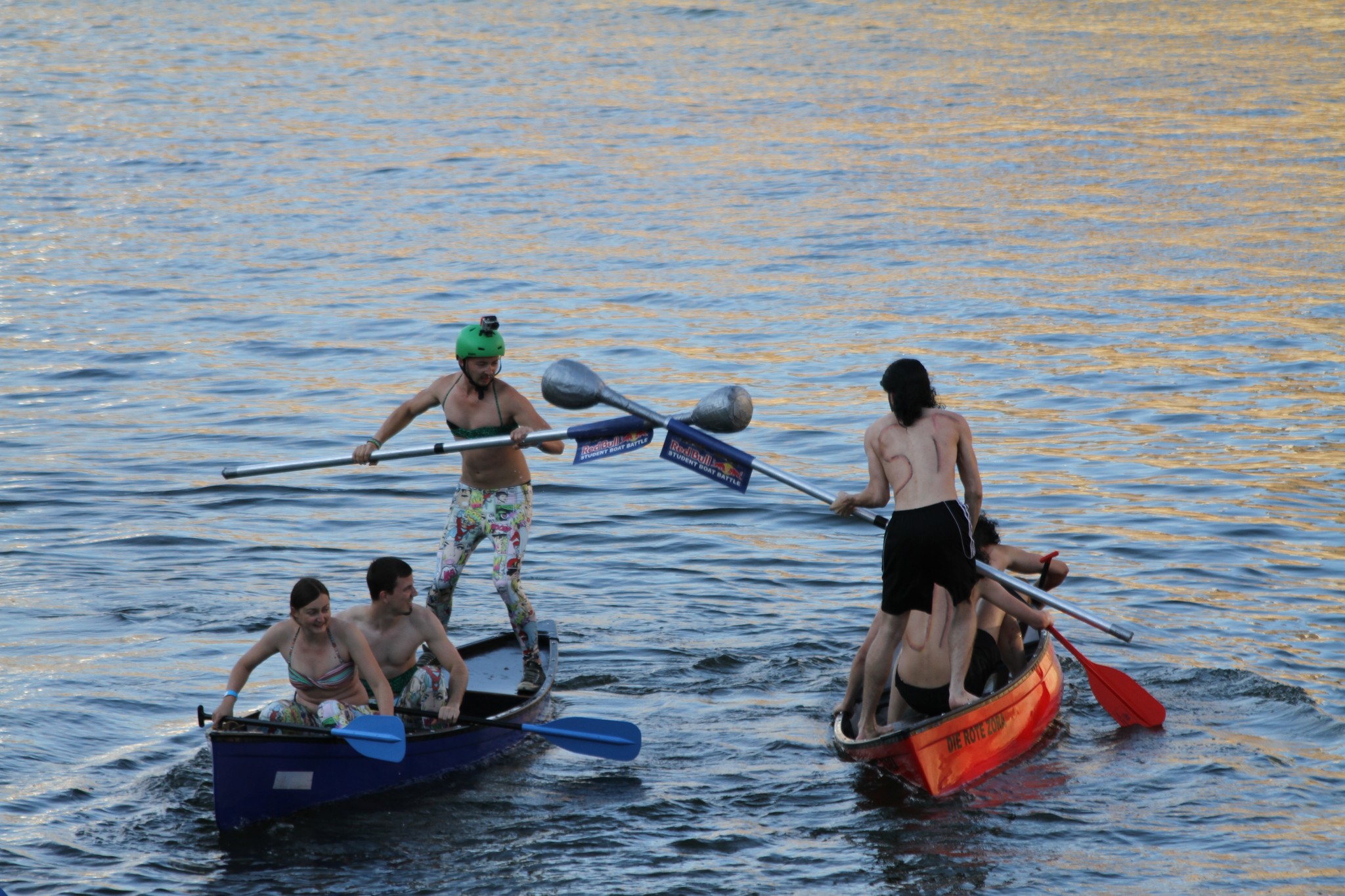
[205,618,562,838]
[830,607,1089,802]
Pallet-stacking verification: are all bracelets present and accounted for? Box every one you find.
[223,690,238,701]
[367,438,382,450]
[531,442,542,448]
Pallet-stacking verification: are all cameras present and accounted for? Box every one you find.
[480,315,500,331]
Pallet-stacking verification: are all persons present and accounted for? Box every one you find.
[828,358,1069,740]
[212,577,394,735]
[330,558,469,732]
[351,325,565,695]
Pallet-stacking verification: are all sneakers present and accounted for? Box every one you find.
[415,644,442,668]
[517,661,546,695]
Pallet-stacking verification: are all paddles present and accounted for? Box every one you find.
[369,698,642,762]
[1005,582,1168,728]
[1033,550,1059,606]
[195,702,409,764]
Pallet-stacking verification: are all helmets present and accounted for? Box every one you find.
[455,324,505,360]
